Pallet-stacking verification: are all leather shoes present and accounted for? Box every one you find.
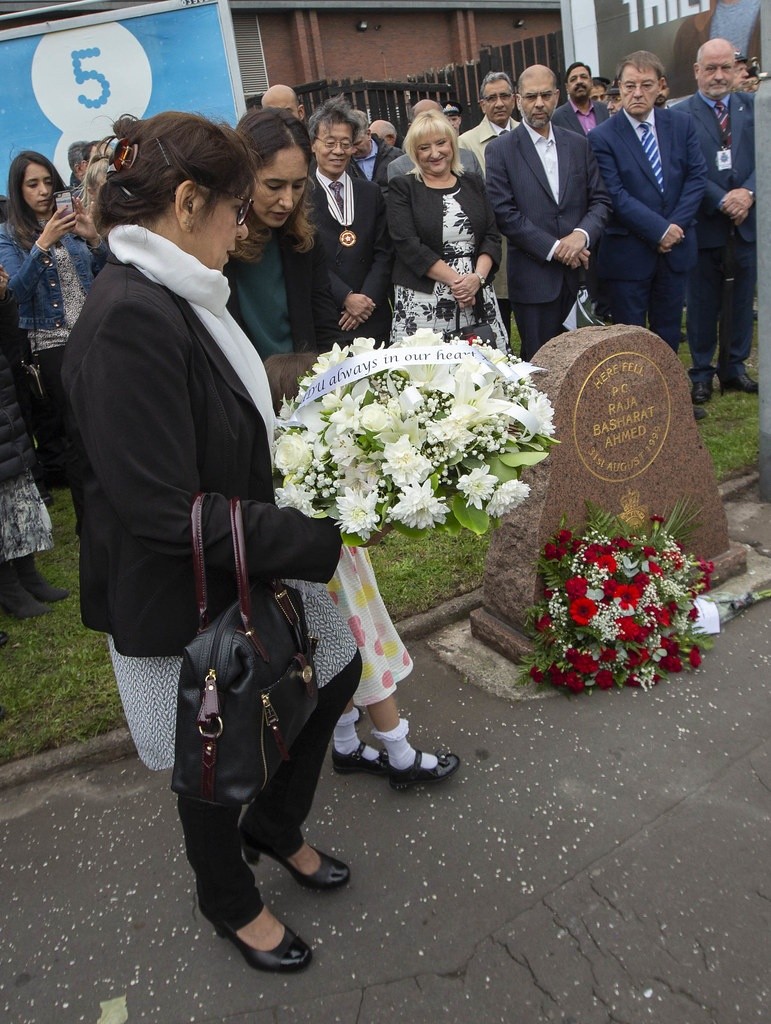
[720,374,759,394]
[691,382,713,405]
[331,741,390,774]
[389,752,461,787]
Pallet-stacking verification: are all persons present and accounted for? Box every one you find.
[62,112,392,974]
[224,38,762,412]
[0,138,119,618]
[262,352,460,790]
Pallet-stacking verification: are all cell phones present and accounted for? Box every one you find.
[53,190,75,227]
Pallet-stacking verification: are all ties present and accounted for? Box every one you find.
[638,121,665,192]
[329,181,345,217]
[713,100,731,151]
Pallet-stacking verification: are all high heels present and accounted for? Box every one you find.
[2,554,68,618]
[203,898,315,973]
[240,816,353,894]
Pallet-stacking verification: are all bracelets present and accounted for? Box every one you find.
[749,190,755,202]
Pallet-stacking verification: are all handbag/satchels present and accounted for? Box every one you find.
[19,362,50,402]
[171,492,318,808]
[443,301,497,349]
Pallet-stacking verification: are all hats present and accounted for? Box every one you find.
[735,52,748,63]
[605,79,622,95]
[441,101,464,117]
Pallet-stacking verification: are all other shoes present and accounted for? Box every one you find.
[693,406,706,420]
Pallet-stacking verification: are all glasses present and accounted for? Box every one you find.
[200,183,256,226]
[521,90,557,102]
[316,137,353,150]
[606,97,622,103]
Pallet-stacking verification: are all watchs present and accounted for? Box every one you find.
[475,272,486,287]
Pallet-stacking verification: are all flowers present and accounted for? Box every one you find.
[272,328,560,548]
[510,493,716,699]
